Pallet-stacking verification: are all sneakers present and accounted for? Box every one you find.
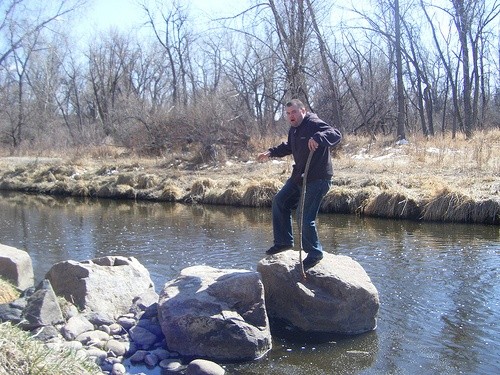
[300,249,323,269]
[265,240,295,254]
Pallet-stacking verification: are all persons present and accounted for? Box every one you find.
[256,99,342,267]
